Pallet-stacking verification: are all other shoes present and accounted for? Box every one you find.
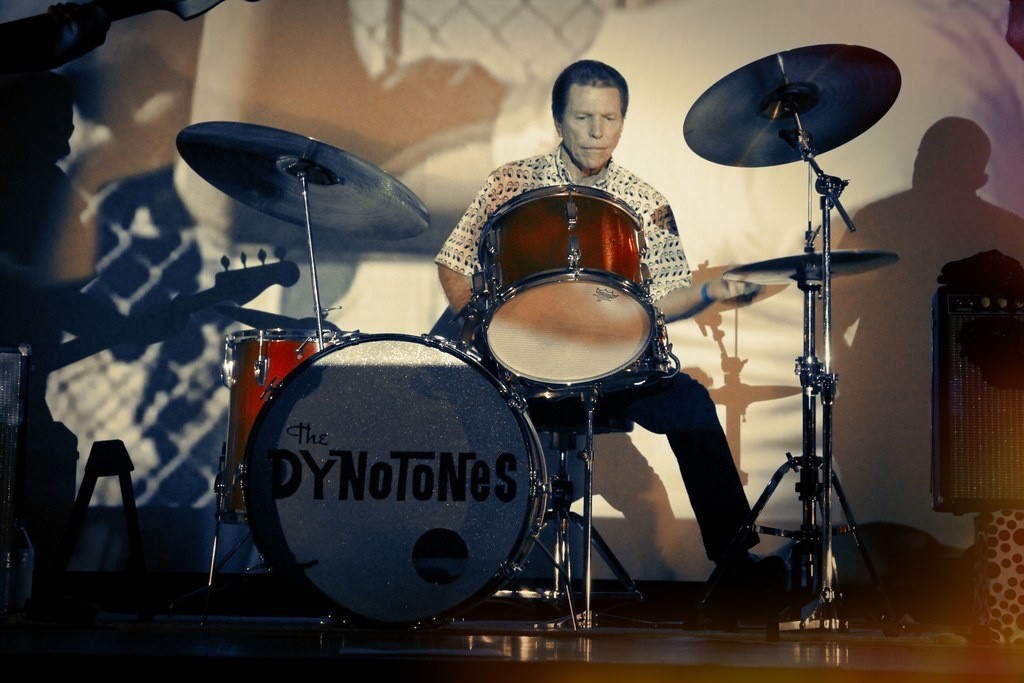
[709,555,788,605]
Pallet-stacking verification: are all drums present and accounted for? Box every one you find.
[210,327,361,526]
[505,305,671,402]
[471,182,659,387]
[239,333,553,637]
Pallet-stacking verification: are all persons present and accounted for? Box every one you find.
[433,60,790,597]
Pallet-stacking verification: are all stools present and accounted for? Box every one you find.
[530,411,649,605]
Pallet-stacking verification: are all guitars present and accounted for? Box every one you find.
[0,0,262,82]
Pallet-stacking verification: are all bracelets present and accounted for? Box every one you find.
[702,284,714,305]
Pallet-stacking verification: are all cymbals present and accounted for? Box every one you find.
[721,247,901,286]
[682,42,904,169]
[174,120,431,242]
